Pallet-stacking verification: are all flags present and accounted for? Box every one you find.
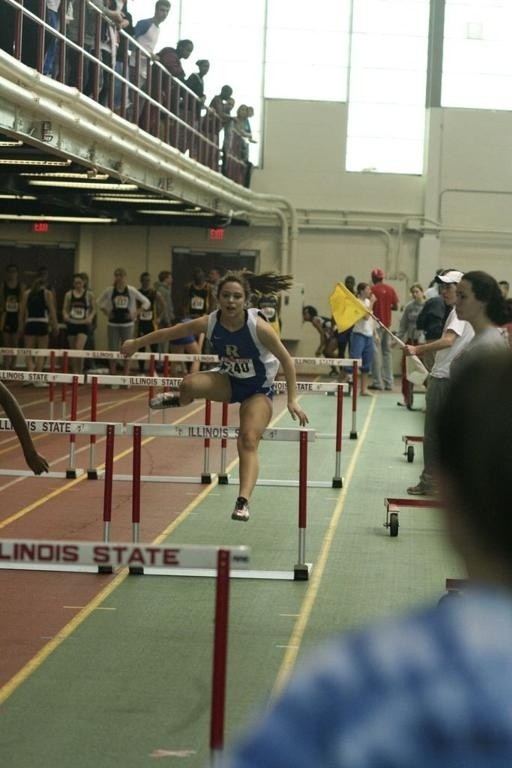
[331,282,369,332]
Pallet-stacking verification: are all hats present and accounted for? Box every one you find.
[435,271,464,283]
[372,269,384,279]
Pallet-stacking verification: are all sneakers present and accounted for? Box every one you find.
[329,370,391,396]
[397,401,408,406]
[407,485,437,495]
[232,497,250,521]
[149,392,194,408]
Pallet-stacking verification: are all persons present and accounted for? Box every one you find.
[120,269,309,522]
[303,305,340,377]
[400,270,475,495]
[339,268,454,411]
[0,265,282,391]
[0,0,257,190]
[0,379,50,476]
[232,342,512,768]
[455,271,512,346]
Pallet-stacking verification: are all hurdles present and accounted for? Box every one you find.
[0,416,123,575]
[86,373,213,484]
[276,356,360,440]
[1,347,59,421]
[61,348,160,424]
[160,352,219,429]
[0,370,85,479]
[219,380,350,488]
[124,420,315,581]
